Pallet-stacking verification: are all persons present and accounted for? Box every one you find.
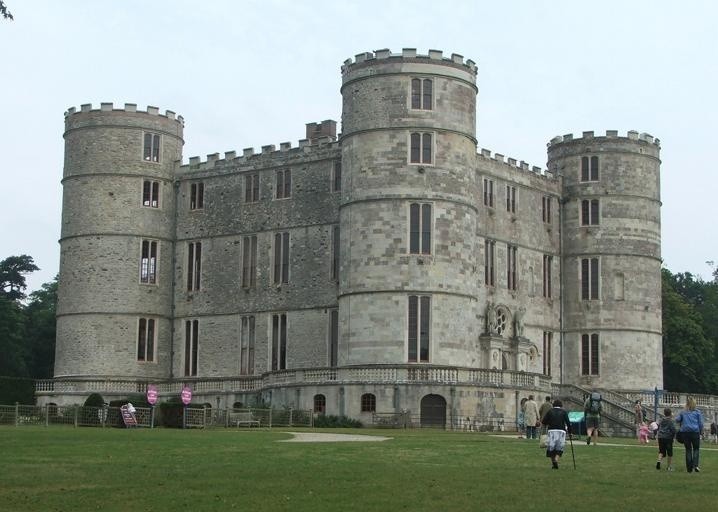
[634,400,647,423]
[538,396,553,435]
[523,394,540,439]
[649,420,659,435]
[674,395,704,472]
[639,421,650,442]
[654,407,677,472]
[541,399,572,469]
[582,392,603,446]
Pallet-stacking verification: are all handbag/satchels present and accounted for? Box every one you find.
[539,423,549,448]
[676,430,686,443]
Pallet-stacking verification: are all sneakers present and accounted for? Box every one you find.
[587,436,591,445]
[552,460,558,469]
[688,466,701,473]
[655,460,661,470]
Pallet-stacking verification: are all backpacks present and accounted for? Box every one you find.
[590,392,602,414]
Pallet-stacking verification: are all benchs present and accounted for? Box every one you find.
[228,412,260,429]
